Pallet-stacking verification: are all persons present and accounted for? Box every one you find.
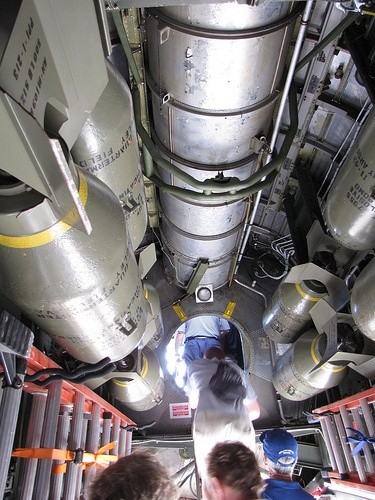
[174,315,231,385]
[182,347,260,500]
[204,441,263,500]
[256,428,316,500]
[86,452,180,500]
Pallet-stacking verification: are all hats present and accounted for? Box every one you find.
[259,429,298,467]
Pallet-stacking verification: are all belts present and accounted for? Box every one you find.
[184,336,220,343]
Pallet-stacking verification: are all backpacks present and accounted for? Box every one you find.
[209,357,246,403]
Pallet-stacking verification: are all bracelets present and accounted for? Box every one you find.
[175,353,180,356]
